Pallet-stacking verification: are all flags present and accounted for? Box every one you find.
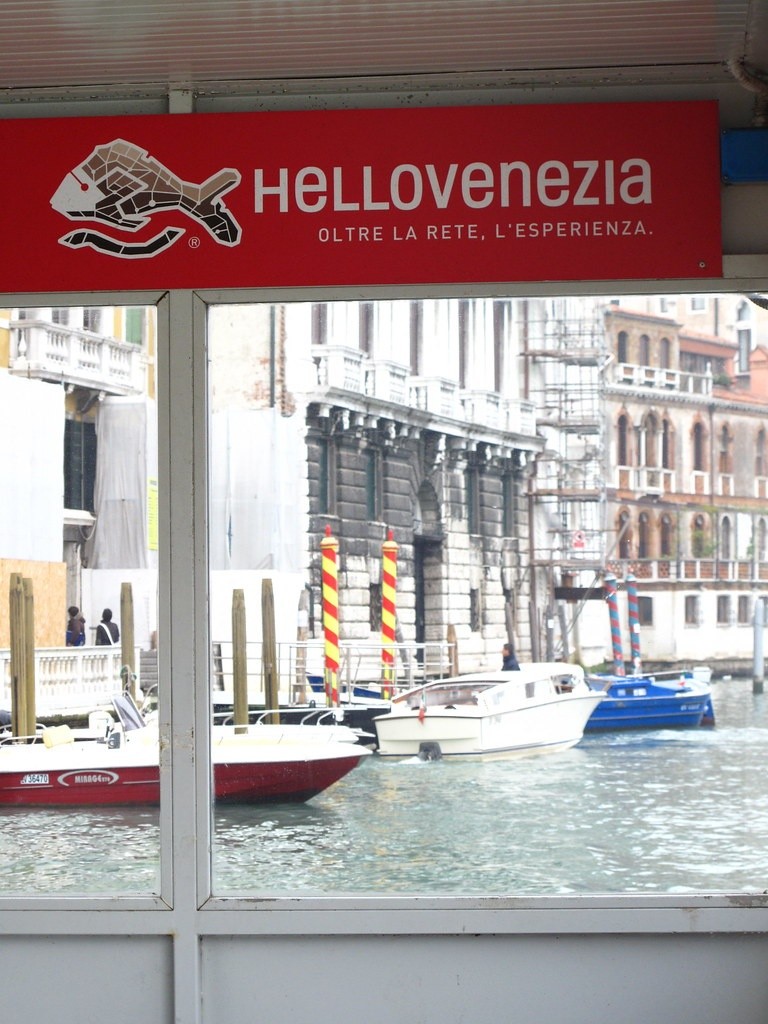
[419,692,425,725]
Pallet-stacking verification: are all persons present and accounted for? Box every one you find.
[501,644,520,671]
[573,535,585,559]
[96,608,119,646]
[66,607,86,646]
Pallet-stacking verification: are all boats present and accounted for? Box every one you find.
[373,662,611,761]
[0,681,376,806]
[586,668,718,734]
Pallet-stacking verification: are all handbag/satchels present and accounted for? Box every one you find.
[67,631,85,646]
